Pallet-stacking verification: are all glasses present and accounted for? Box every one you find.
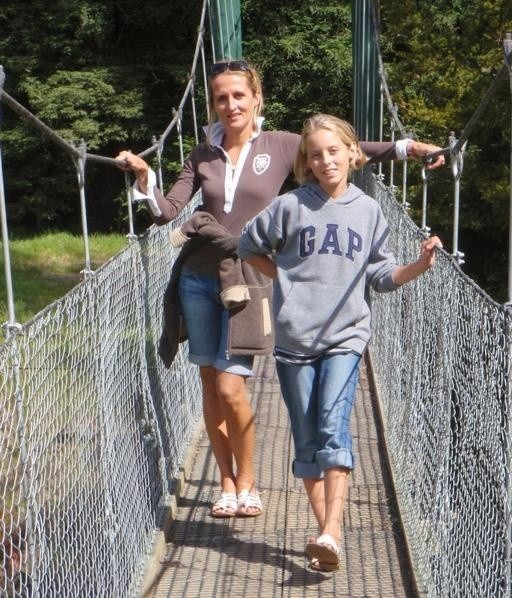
[209,60,252,76]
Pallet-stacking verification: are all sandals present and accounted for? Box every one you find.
[237,491,264,519]
[305,532,345,582]
[210,491,239,519]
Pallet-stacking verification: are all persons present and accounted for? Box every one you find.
[239,109,445,580]
[116,57,446,518]
